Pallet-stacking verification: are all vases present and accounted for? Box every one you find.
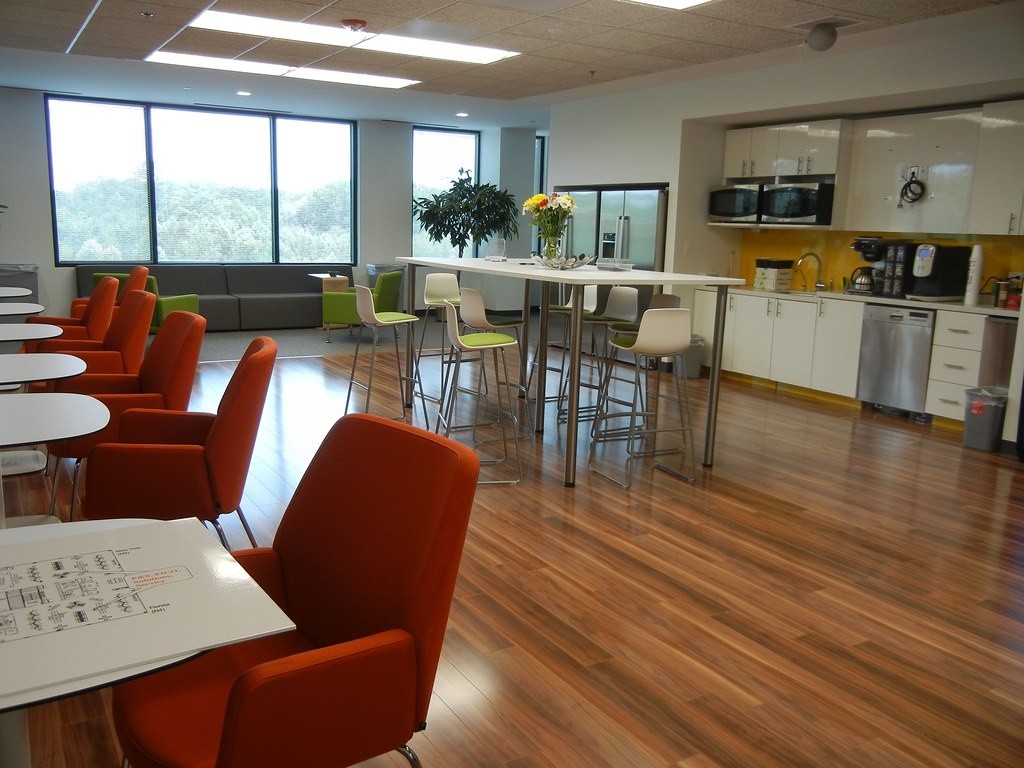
[540,236,563,260]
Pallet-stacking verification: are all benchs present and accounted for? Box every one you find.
[223,264,354,330]
[75,265,242,332]
[90,273,198,333]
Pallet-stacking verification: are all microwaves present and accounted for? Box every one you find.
[706,184,759,222]
[760,182,832,224]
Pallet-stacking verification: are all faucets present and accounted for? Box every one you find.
[797,251,834,292]
[794,269,807,291]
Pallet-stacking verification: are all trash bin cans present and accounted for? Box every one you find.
[367,263,407,312]
[0,263,39,354]
[963,386,1008,452]
[672,334,705,379]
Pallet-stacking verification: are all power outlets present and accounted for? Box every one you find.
[910,167,918,181]
[1008,272,1023,282]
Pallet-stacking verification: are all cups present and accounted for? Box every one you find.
[963,244,983,306]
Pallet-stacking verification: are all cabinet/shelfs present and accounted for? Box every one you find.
[924,309,1005,432]
[968,99,1024,235]
[706,118,855,231]
[692,289,865,409]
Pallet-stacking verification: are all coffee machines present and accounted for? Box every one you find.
[845,236,888,296]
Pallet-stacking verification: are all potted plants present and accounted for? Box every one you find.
[408,166,521,321]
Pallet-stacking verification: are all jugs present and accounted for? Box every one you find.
[851,266,876,290]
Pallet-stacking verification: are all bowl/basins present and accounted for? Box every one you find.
[328,271,339,277]
[595,257,635,271]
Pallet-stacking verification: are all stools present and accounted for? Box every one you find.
[528,283,697,490]
[345,274,535,485]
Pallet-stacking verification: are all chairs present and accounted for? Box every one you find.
[17,265,482,767]
[323,272,403,346]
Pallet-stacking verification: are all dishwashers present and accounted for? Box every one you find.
[857,304,934,414]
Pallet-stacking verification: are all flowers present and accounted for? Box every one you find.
[522,192,578,258]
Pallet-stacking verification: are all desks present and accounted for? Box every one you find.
[0,286,214,713]
[307,273,349,330]
[396,257,746,486]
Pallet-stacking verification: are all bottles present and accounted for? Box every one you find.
[994,279,1010,307]
